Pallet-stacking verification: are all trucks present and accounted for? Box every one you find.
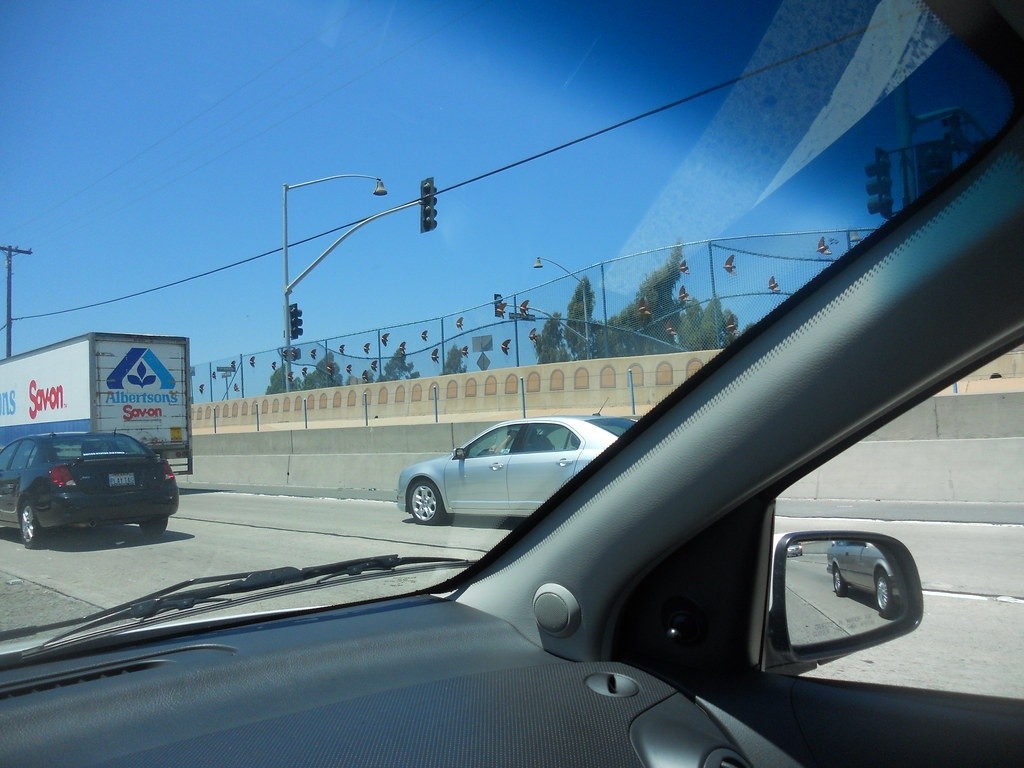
[0,331,196,478]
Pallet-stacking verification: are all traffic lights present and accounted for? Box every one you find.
[494,294,505,318]
[863,149,890,215]
[942,111,961,150]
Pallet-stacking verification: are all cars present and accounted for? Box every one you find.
[824,540,906,618]
[0,432,178,551]
[394,410,647,527]
[786,539,803,559]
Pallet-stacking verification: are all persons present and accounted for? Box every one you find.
[494,425,538,455]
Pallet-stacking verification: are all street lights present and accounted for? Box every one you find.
[283,174,390,391]
[533,257,592,360]
